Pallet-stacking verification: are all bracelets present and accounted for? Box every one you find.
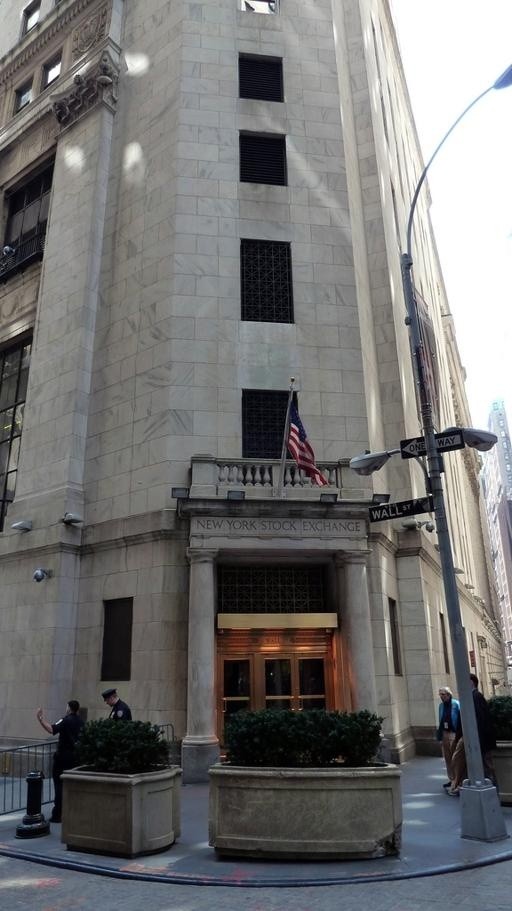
[39,716,43,722]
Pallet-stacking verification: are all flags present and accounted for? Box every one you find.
[287,401,327,487]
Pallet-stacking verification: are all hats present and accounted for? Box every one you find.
[100,688,117,701]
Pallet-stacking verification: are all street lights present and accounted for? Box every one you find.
[350,58,512,843]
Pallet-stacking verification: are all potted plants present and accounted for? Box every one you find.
[474,687,512,806]
[54,708,186,863]
[203,702,414,863]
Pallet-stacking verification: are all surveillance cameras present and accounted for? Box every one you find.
[426,522,435,532]
[31,569,44,583]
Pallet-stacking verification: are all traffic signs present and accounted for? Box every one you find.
[395,430,463,465]
[364,495,437,525]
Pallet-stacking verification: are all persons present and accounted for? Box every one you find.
[37,701,80,823]
[448,673,499,799]
[101,689,132,724]
[436,687,462,788]
